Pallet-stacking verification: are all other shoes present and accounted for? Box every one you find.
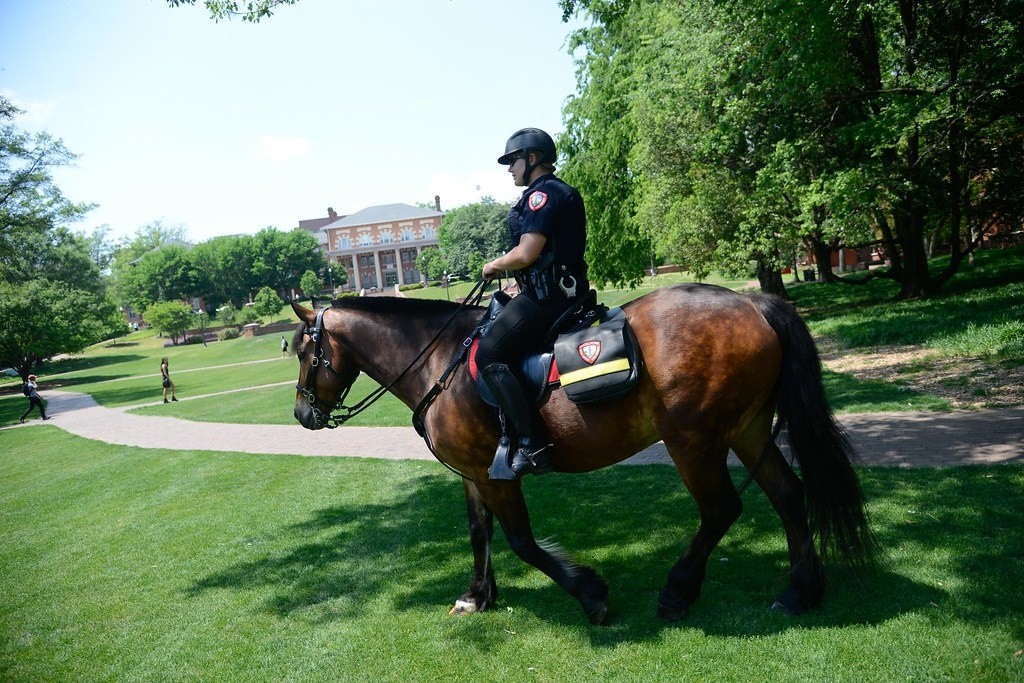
[19,418,25,423]
[43,417,53,420]
[172,396,178,401]
[163,398,171,403]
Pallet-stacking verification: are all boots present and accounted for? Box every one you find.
[481,364,550,472]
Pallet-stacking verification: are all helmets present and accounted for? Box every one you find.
[497,128,557,163]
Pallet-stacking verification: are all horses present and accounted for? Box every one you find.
[288,282,889,629]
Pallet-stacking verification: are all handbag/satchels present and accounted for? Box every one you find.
[162,379,170,388]
[553,317,640,408]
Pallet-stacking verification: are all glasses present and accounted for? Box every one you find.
[509,156,524,166]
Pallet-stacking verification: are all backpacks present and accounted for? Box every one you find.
[286,341,289,347]
[22,382,33,397]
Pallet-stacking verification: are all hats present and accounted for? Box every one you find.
[29,375,37,378]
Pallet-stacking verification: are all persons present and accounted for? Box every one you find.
[476,128,588,477]
[160,356,179,403]
[18,374,51,423]
[281,335,289,359]
